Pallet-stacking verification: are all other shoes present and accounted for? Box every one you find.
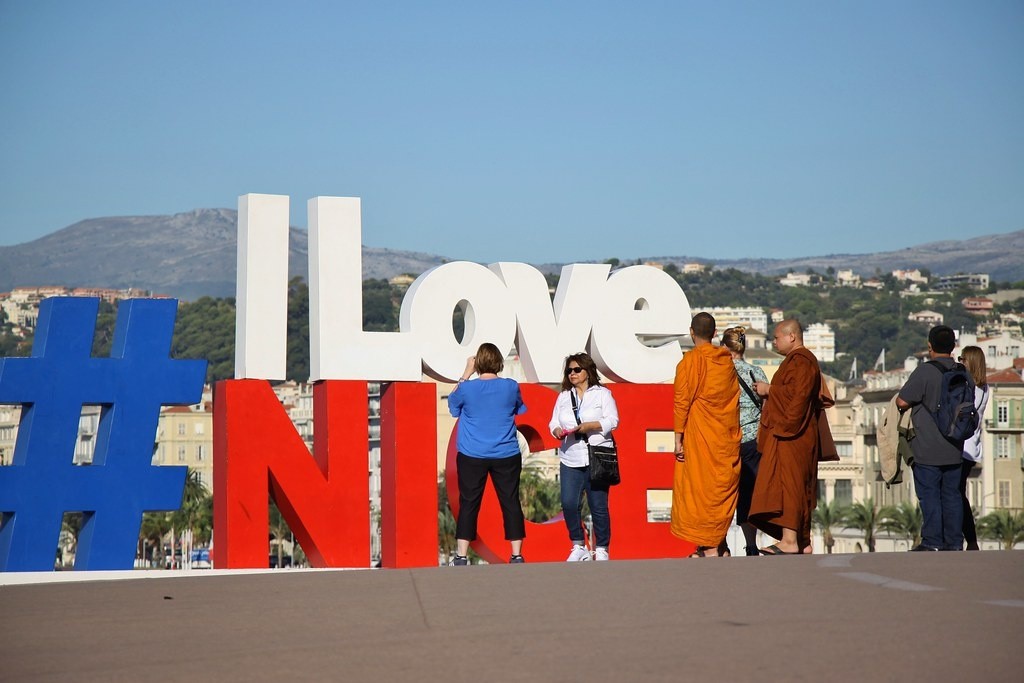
[509,555,525,563]
[907,544,942,551]
[689,543,730,558]
[939,543,964,551]
[966,543,979,550]
[453,555,467,565]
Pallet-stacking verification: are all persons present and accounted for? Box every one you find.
[895,325,975,551]
[958,346,989,551]
[752,319,840,555]
[549,351,619,561]
[721,326,769,555]
[671,312,743,557]
[448,343,528,565]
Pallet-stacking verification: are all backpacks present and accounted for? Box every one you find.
[917,361,979,440]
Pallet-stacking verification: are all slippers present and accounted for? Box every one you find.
[757,544,785,554]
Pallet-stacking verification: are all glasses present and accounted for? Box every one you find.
[958,356,963,362]
[566,366,582,375]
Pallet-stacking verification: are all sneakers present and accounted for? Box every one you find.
[590,547,609,560]
[566,544,590,562]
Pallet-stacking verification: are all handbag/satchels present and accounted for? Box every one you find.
[586,443,620,485]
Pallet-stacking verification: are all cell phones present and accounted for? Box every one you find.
[750,370,756,384]
